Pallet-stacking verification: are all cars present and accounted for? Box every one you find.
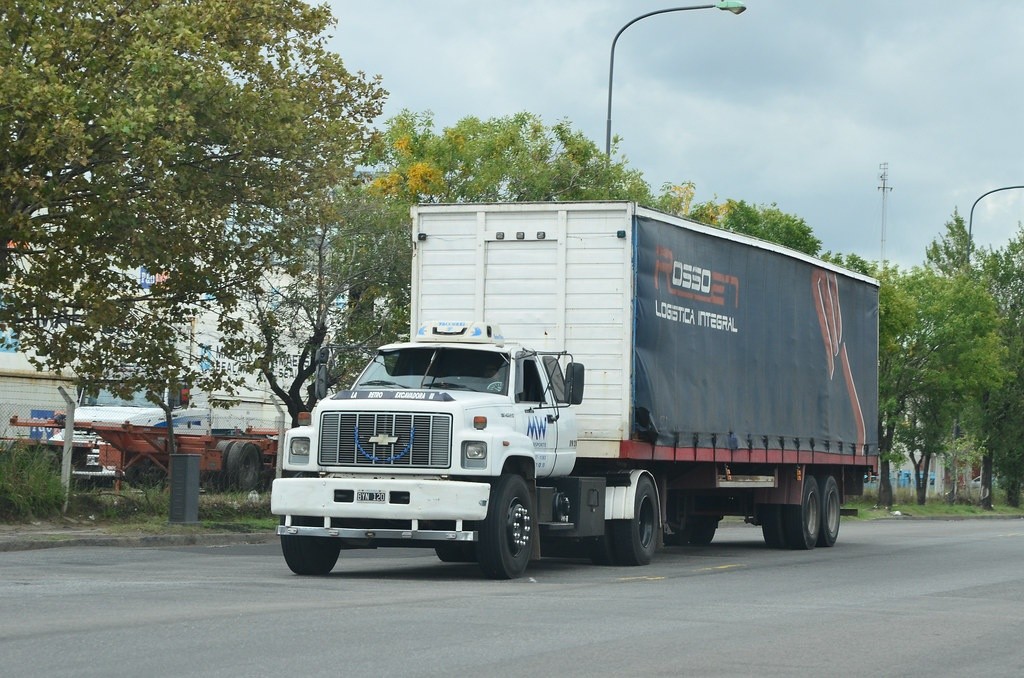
[971,475,999,487]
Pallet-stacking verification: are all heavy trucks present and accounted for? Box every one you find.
[268,197,882,581]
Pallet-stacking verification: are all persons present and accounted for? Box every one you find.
[478,358,501,380]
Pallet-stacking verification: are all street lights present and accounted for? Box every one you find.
[605,1,748,174]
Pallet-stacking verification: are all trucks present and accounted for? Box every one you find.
[14,391,309,494]
